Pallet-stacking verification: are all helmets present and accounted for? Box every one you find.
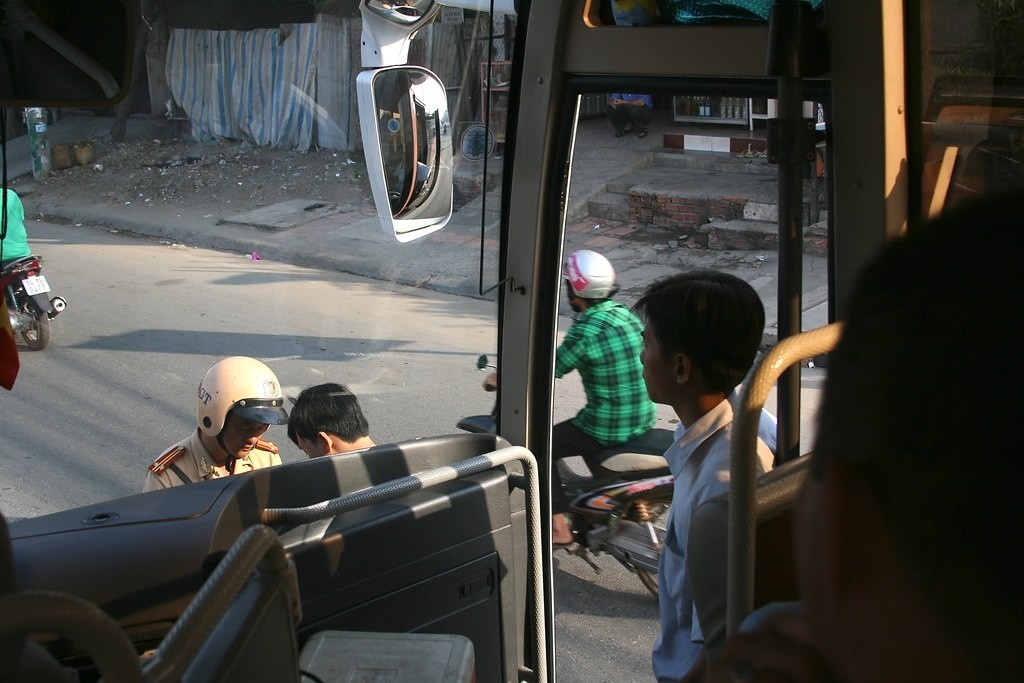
[564,250,616,298]
[196,356,289,438]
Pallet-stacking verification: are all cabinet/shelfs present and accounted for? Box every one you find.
[480,61,511,143]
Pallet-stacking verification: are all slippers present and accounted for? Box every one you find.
[638,128,648,137]
[615,127,634,137]
[553,542,573,549]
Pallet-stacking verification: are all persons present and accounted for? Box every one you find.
[0,187,32,272]
[483,250,658,551]
[141,356,289,493]
[287,383,376,459]
[607,93,653,138]
[387,145,429,200]
[630,182,1024,683]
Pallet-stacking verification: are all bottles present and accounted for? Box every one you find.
[720,97,749,119]
[680,95,712,117]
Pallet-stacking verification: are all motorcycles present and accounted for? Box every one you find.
[456,355,676,602]
[3,254,66,351]
